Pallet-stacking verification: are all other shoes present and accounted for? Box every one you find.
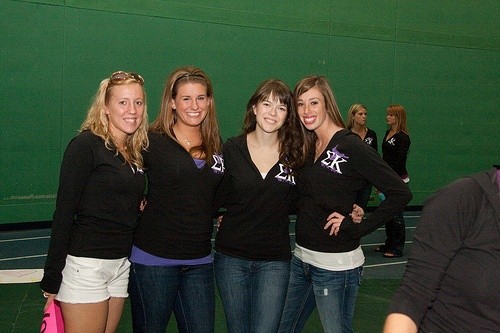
[376,245,387,252]
[383,250,404,257]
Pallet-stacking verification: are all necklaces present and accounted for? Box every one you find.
[172,128,202,146]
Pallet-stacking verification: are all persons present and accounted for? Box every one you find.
[130,66,225,333]
[216,75,414,333]
[374,105,411,257]
[140,79,365,333]
[40,70,149,333]
[383,165,500,332]
[346,104,378,211]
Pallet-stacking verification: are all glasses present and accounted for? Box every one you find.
[106,71,144,90]
